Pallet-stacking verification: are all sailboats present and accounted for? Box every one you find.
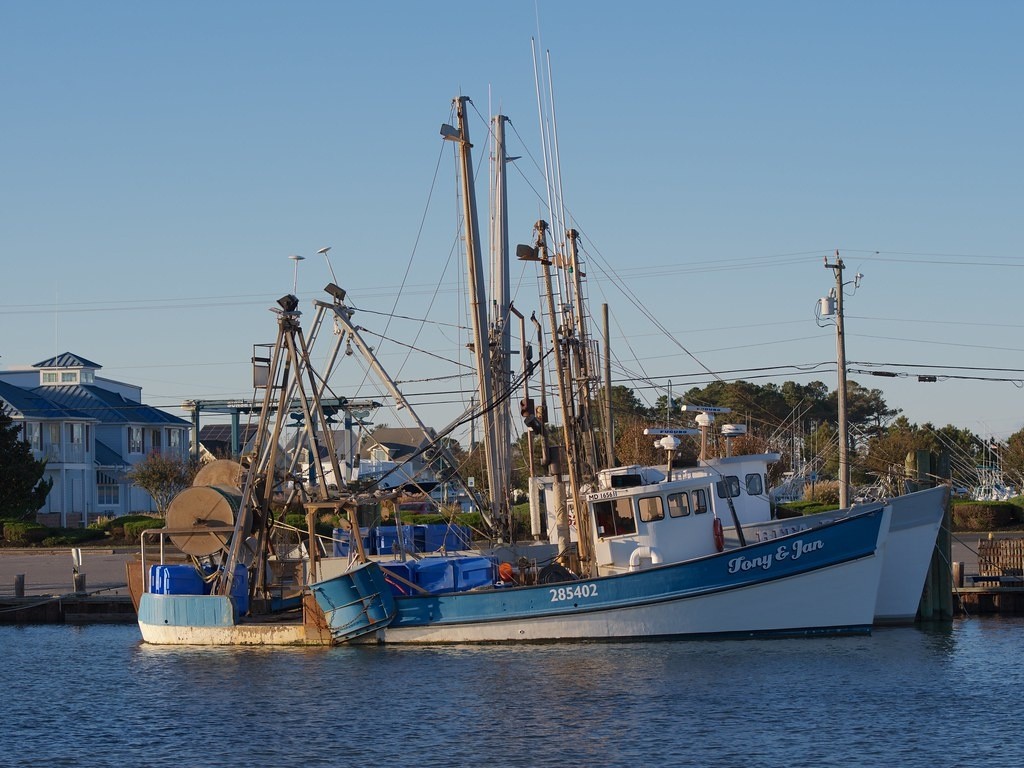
[124,35,958,647]
